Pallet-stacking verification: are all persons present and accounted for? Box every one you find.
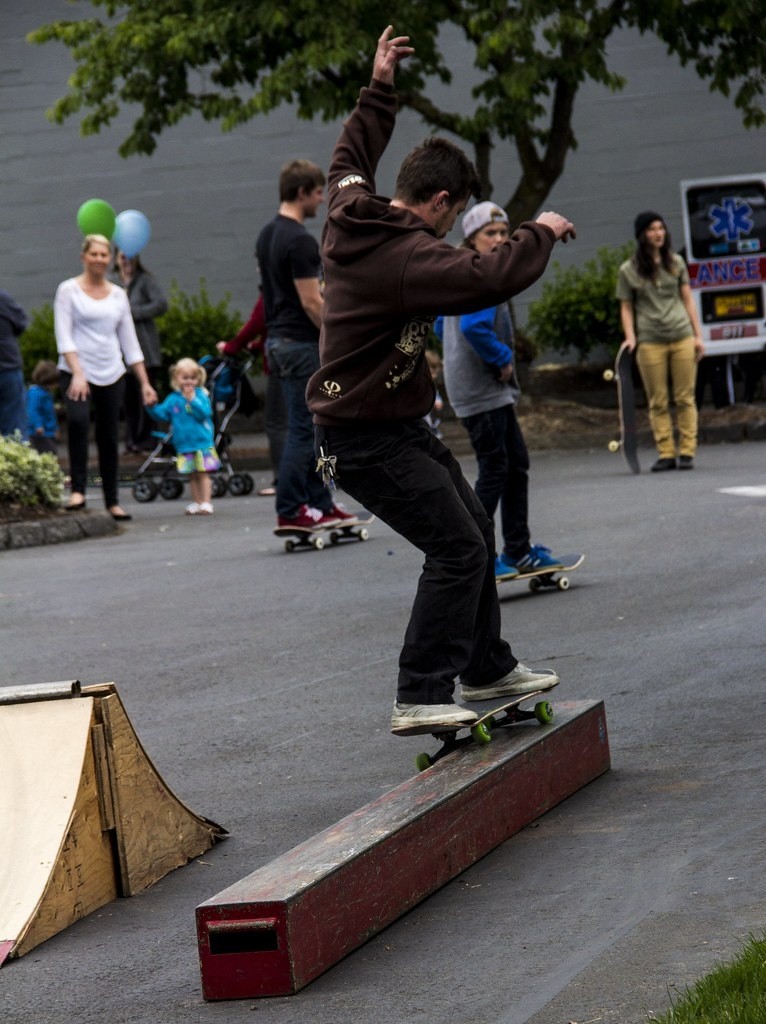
[305,26,576,730]
[215,253,288,496]
[52,234,158,522]
[618,209,704,473]
[144,358,222,515]
[254,159,358,531]
[432,201,562,579]
[0,292,31,448]
[22,359,63,460]
[418,343,447,459]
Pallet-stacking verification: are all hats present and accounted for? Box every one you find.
[635,211,663,239]
[461,201,509,238]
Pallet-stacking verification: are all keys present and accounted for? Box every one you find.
[317,455,340,492]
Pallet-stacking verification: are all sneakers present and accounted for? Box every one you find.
[391,696,479,727]
[495,556,519,580]
[460,662,560,701]
[500,544,564,573]
[278,504,341,529]
[324,498,359,522]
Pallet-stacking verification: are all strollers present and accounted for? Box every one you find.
[132,354,255,502]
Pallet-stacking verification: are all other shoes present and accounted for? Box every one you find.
[200,502,214,514]
[185,503,200,514]
[652,458,676,471]
[66,501,86,511]
[680,455,695,469]
[113,514,133,521]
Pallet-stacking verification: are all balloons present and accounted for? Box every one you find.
[113,209,152,258]
[77,197,117,241]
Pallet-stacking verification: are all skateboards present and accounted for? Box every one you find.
[495,552,585,591]
[274,511,376,553]
[602,340,642,475]
[390,667,559,774]
